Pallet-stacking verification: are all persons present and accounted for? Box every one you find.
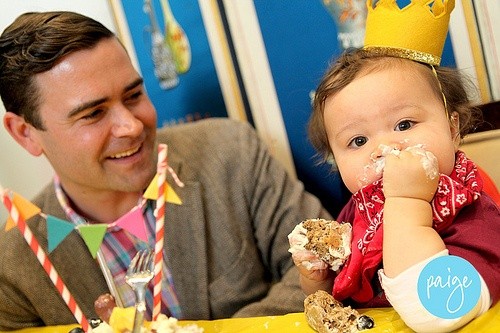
[292,45,500,333]
[0,11,339,330]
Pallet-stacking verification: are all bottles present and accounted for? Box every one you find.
[159,1,191,75]
[144,1,179,89]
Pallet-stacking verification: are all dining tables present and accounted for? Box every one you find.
[1,303,500,333]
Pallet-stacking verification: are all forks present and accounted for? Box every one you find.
[124,249,155,333]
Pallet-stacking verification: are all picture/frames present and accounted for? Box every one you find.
[107,0,257,136]
[237,0,499,220]
